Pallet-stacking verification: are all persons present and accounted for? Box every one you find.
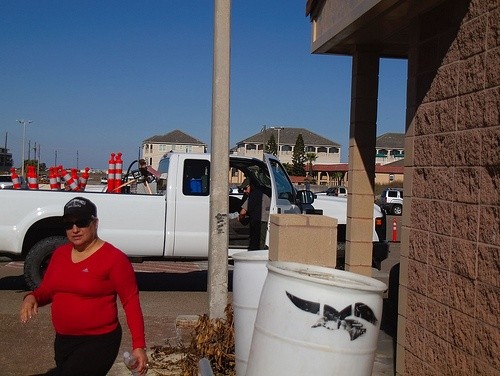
[238,179,271,250]
[21,196,148,376]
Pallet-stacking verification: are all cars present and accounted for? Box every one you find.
[0,175,14,189]
[100,177,108,185]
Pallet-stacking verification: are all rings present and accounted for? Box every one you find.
[145,364,149,368]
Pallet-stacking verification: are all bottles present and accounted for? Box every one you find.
[123,351,142,376]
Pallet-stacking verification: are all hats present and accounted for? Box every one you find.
[62,197,98,221]
[240,177,250,188]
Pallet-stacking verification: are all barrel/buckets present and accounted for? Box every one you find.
[230,250,388,376]
[185,179,203,193]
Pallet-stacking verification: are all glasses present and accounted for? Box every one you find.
[64,219,96,231]
[243,186,250,190]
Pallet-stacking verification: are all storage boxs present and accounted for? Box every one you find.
[268,214,338,268]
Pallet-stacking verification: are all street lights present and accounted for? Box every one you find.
[15,119,33,177]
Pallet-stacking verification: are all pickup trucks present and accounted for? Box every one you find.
[0,150,388,291]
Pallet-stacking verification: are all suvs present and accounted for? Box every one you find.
[379,187,403,216]
